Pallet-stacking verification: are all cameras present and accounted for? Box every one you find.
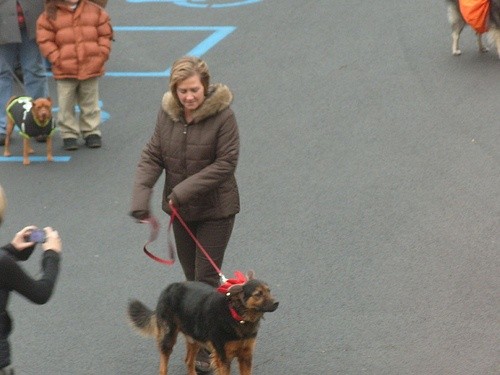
[30,229,47,243]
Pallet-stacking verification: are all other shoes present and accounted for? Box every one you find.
[195,347,213,375]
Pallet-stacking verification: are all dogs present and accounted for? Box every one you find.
[4,94,55,167]
[441,0,500,56]
[126,268,280,375]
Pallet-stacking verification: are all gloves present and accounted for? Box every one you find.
[133,211,150,222]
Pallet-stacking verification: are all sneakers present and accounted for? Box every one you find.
[86,134,101,147]
[63,138,78,151]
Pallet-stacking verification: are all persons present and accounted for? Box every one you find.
[128,57,240,374]
[0,0,49,146]
[35,0,114,149]
[0,187,62,375]
